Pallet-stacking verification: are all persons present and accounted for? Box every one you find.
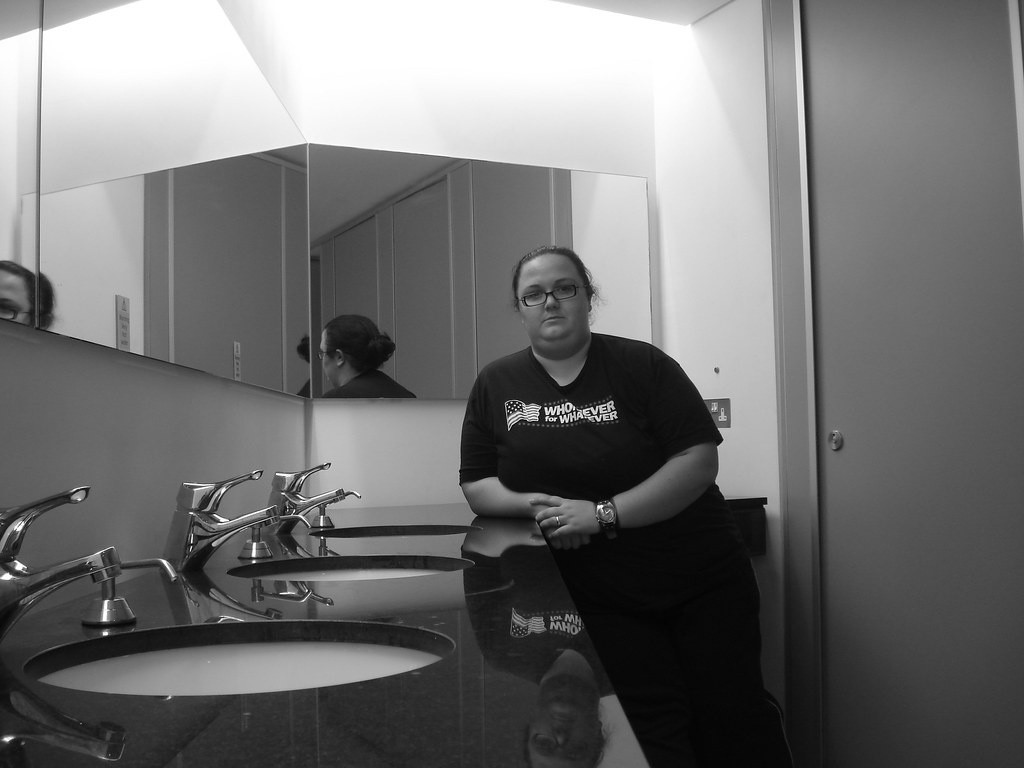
[0,260,54,330]
[458,245,795,767]
[321,314,416,398]
[461,516,616,768]
[296,336,310,398]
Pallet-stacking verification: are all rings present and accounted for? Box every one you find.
[556,516,561,526]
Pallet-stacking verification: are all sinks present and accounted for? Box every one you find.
[21,618,456,699]
[226,553,476,582]
[309,524,483,539]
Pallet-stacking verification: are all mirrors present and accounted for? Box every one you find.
[308,141,652,401]
[0,143,308,401]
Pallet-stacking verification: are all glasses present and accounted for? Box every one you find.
[0,304,30,321]
[517,284,586,307]
[318,350,333,360]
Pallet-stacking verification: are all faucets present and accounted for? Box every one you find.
[250,577,336,608]
[266,462,346,535]
[309,489,362,527]
[180,568,283,623]
[0,663,128,762]
[161,469,281,572]
[0,485,123,643]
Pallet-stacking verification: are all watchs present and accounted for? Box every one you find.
[595,499,617,531]
